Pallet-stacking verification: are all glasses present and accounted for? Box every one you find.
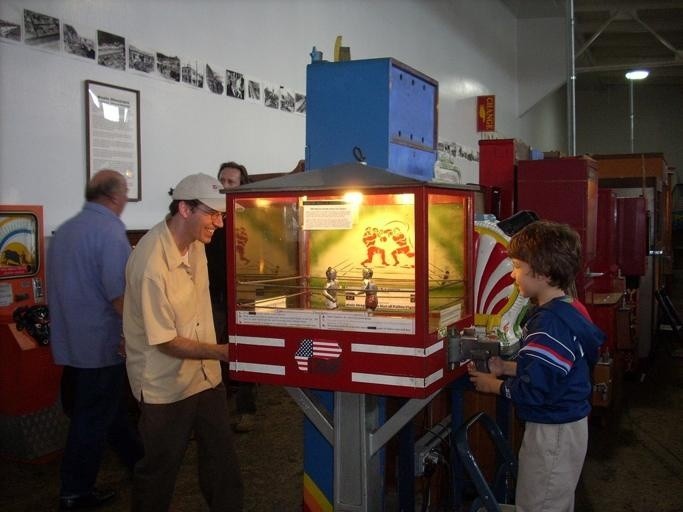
[196,206,228,220]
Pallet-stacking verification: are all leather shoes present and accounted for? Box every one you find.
[59,484,118,511]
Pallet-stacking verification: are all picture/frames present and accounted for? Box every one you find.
[84,79,143,204]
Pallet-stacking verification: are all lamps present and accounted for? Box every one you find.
[625,70,649,81]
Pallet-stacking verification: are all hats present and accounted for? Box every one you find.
[168,174,227,211]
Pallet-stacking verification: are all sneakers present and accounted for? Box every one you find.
[235,400,261,432]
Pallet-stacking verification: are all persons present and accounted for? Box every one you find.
[121,175,246,512]
[320,267,342,310]
[203,157,258,431]
[353,267,378,311]
[467,220,607,512]
[43,170,137,512]
